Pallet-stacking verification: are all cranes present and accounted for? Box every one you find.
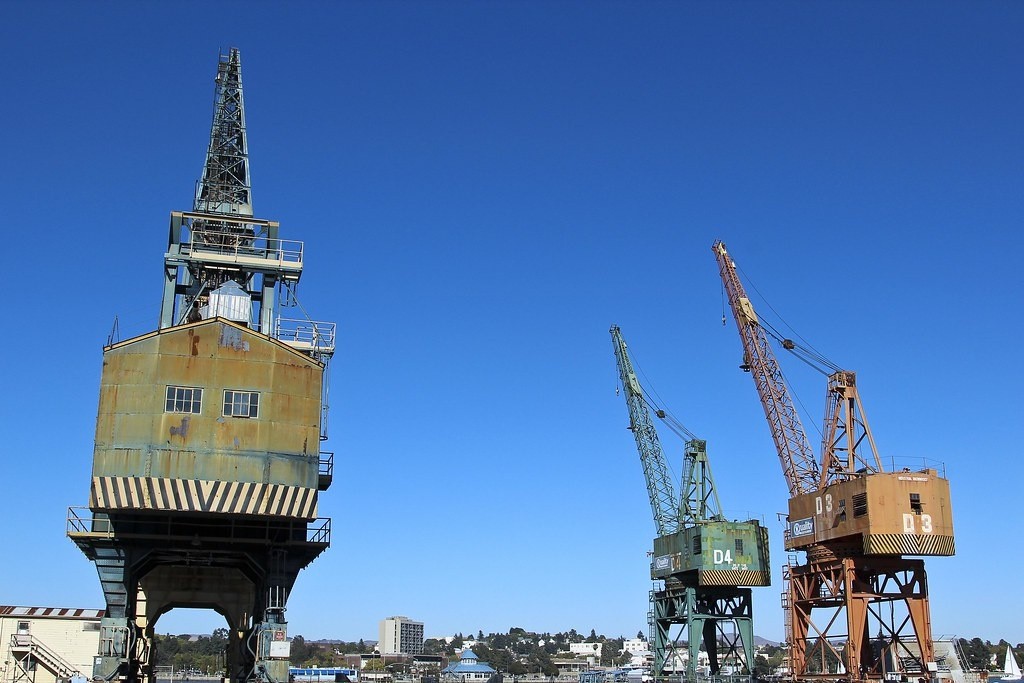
[709,234,958,683]
[65,44,340,682]
[609,324,773,683]
